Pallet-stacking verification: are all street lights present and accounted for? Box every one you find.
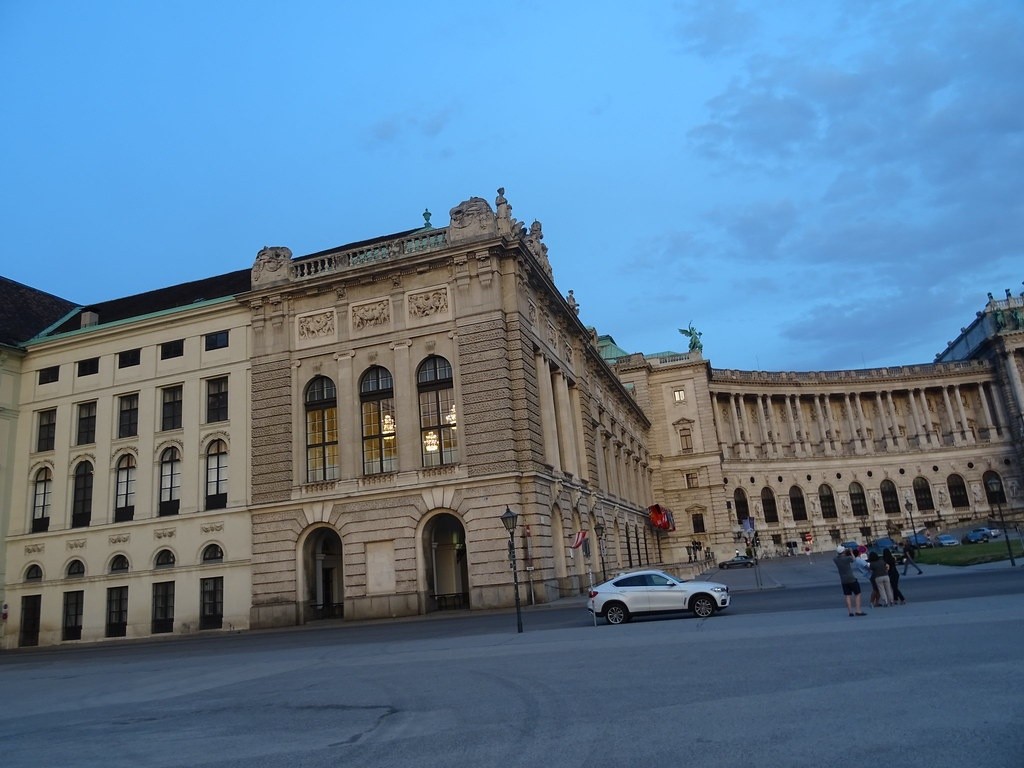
[858,514,869,545]
[986,478,1016,566]
[904,499,921,555]
[499,504,524,634]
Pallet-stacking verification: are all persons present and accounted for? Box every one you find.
[833,545,866,615]
[883,548,906,605]
[495,187,508,218]
[688,322,703,351]
[853,550,880,608]
[567,289,579,313]
[868,552,894,607]
[899,543,923,575]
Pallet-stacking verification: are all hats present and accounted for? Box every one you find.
[837,545,845,553]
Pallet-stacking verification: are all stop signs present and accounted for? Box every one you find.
[805,534,813,541]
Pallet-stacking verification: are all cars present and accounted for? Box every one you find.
[904,534,933,550]
[841,540,860,558]
[871,538,898,551]
[972,527,1001,538]
[586,569,732,625]
[933,535,960,547]
[719,556,759,570]
[962,532,989,545]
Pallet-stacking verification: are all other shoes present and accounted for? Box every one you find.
[918,571,923,574]
[849,612,853,616]
[856,612,867,616]
[901,573,906,575]
